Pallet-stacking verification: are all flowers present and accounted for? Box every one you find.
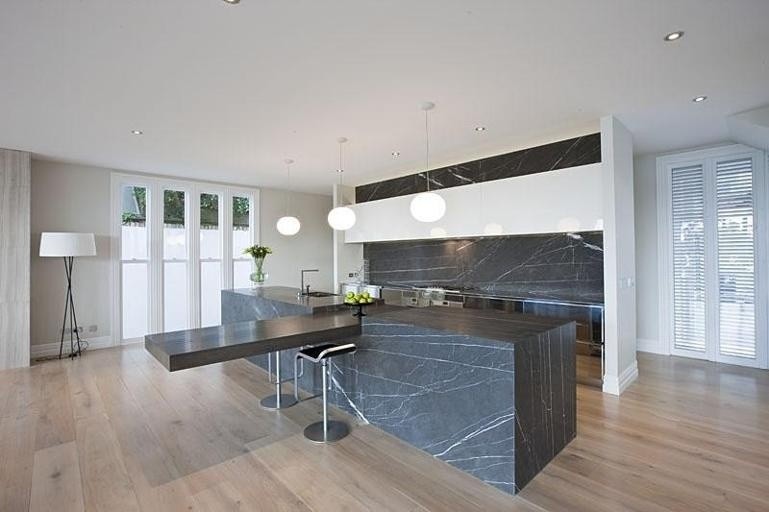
[242,245,272,271]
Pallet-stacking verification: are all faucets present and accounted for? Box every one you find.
[301,269,319,294]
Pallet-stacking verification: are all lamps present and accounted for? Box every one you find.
[411,101,446,221]
[277,158,301,235]
[328,136,356,230]
[40,232,95,360]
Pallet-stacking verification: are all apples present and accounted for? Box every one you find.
[343,291,374,305]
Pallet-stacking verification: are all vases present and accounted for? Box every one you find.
[249,257,267,290]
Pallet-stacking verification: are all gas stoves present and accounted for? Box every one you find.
[413,284,462,294]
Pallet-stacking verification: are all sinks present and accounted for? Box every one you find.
[303,291,339,297]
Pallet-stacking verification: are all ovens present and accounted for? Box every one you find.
[405,291,466,309]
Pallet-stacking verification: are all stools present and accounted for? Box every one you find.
[259,346,303,412]
[294,344,357,443]
